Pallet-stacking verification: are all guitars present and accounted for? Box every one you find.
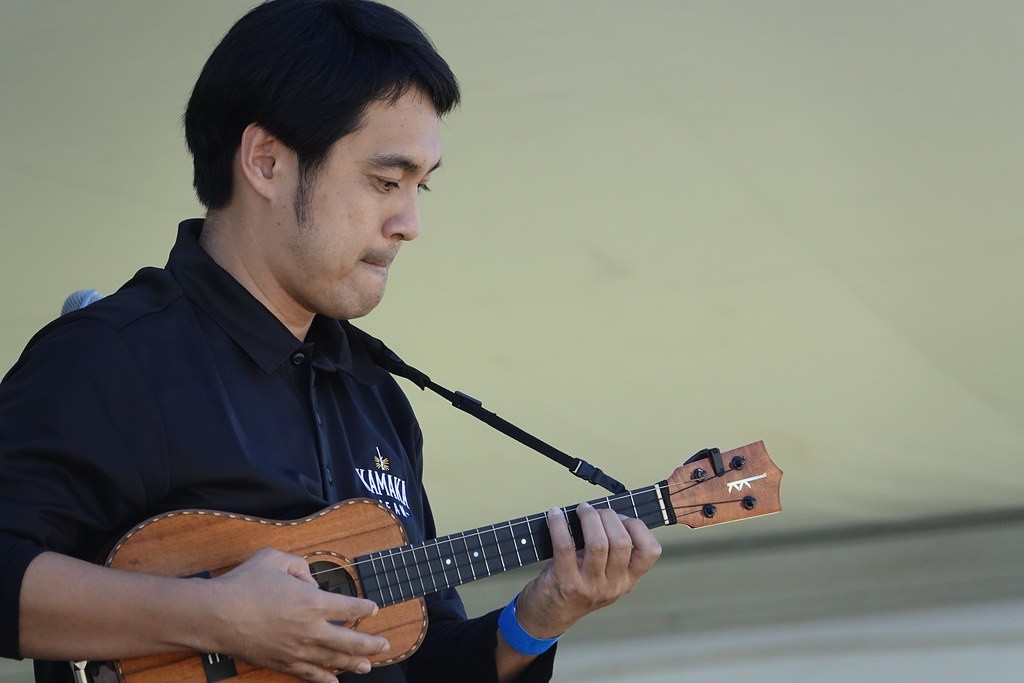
[102,439,785,683]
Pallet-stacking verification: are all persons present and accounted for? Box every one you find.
[1,1,661,683]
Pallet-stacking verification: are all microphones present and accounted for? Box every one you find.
[61,290,104,315]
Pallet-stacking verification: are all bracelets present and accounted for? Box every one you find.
[498,592,565,656]
